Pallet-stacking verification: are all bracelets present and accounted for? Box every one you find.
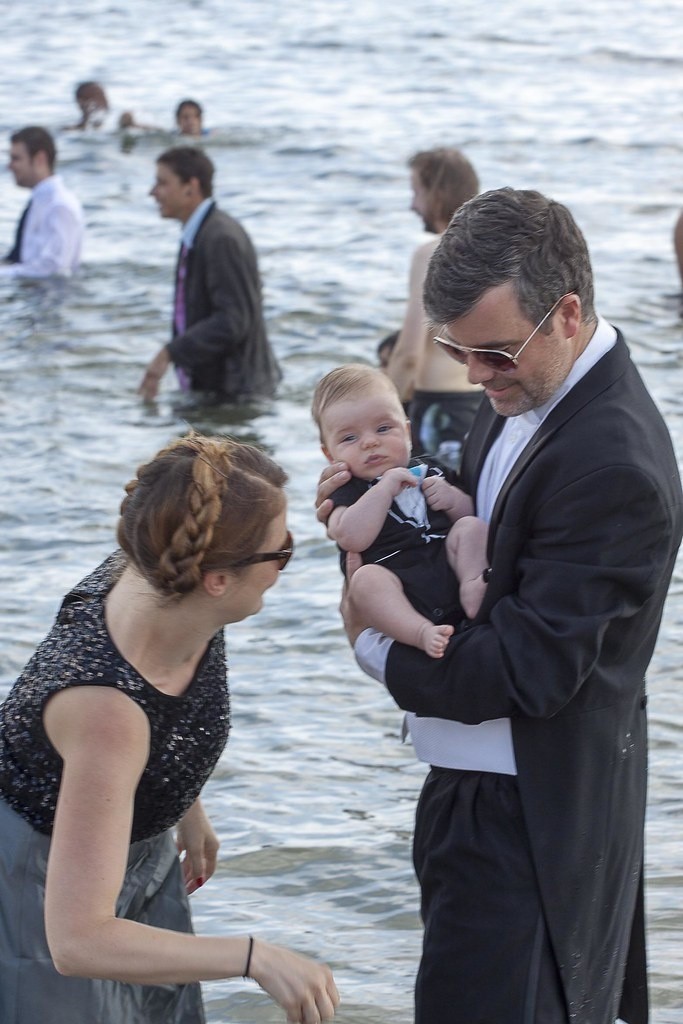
[242,935,253,977]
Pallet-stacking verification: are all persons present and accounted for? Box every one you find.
[58,82,204,143]
[316,186,683,1024]
[0,432,340,1024]
[136,146,285,403]
[0,125,85,280]
[311,363,490,658]
[373,150,496,500]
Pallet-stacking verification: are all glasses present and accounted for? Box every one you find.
[433,289,577,373]
[233,528,293,571]
